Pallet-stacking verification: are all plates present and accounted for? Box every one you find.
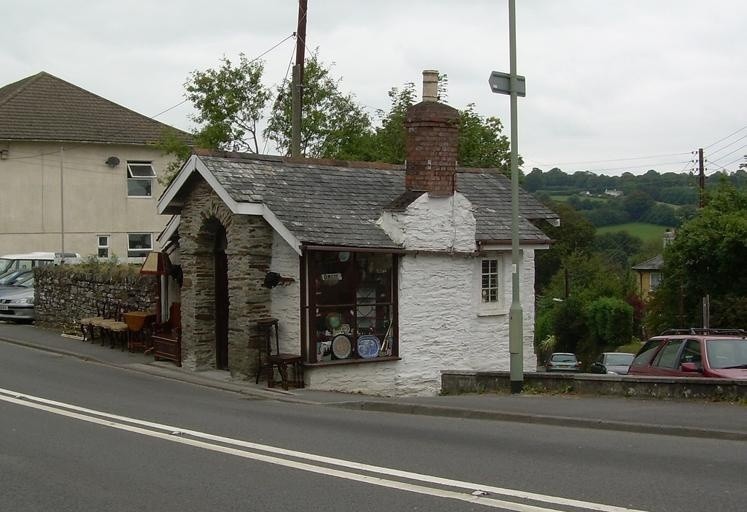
[332,334,381,359]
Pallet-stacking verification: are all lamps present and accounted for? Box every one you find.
[141,251,175,323]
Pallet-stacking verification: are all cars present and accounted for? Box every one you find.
[543,352,637,375]
[0,269,34,324]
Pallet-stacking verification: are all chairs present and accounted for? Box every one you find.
[151,302,182,366]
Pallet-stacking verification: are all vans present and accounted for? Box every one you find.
[0,251,89,284]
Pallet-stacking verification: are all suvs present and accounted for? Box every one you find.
[627,328,747,378]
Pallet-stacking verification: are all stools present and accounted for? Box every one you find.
[79,317,128,352]
[266,353,304,391]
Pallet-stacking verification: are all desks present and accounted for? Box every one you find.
[123,311,157,355]
